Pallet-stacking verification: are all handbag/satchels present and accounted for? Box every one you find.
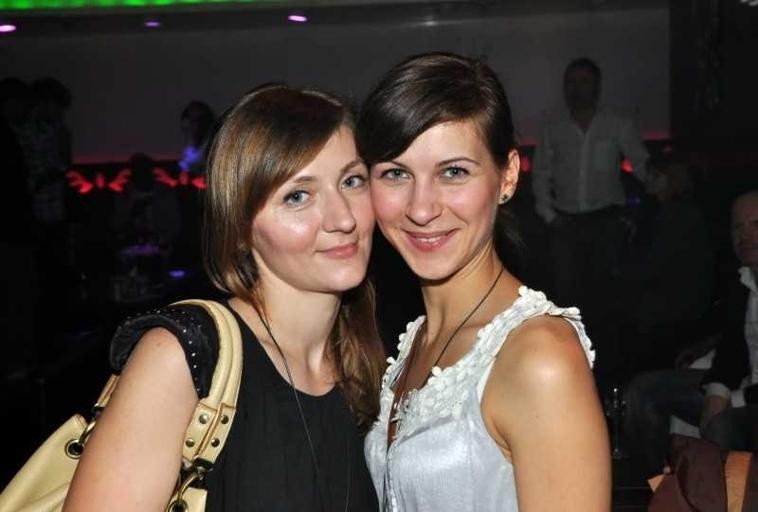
[0,414,208,512]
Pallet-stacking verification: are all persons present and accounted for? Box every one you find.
[624,191,758,492]
[62,83,396,512]
[532,58,652,223]
[0,77,73,378]
[362,53,612,511]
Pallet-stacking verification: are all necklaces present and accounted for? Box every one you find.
[247,289,354,512]
[386,263,507,452]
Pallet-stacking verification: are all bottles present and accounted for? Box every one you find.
[604,383,628,460]
